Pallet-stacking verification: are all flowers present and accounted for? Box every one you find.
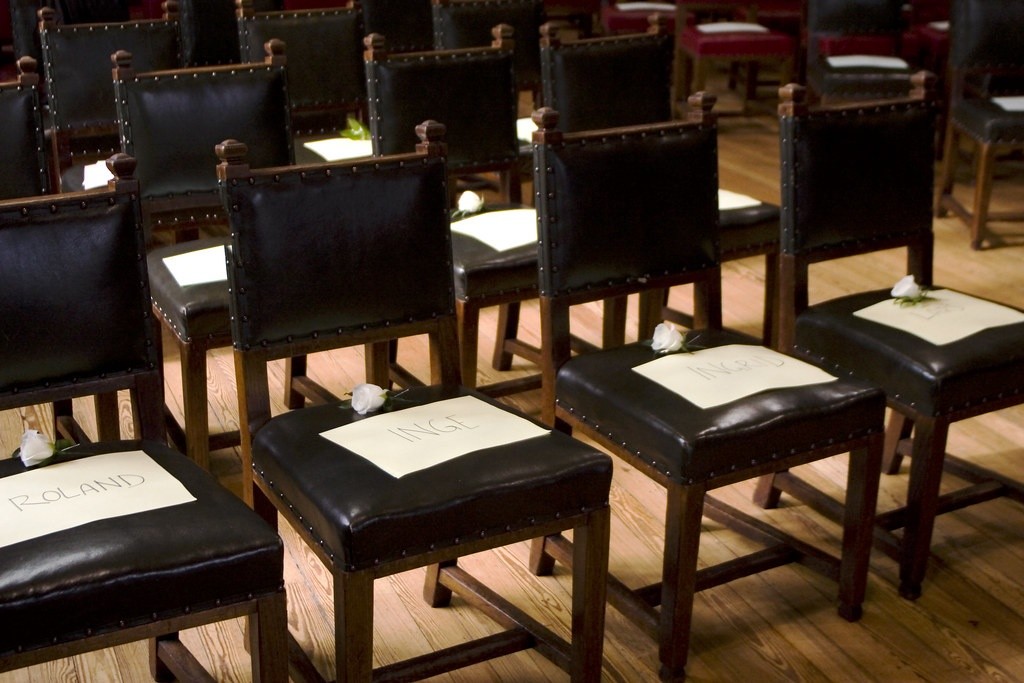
[650,320,700,355]
[451,190,494,220]
[12,429,71,468]
[891,275,944,309]
[338,384,416,415]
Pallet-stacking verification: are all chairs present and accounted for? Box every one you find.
[934,0,1024,252]
[363,24,627,401]
[596,0,953,120]
[532,89,886,683]
[0,0,602,200]
[112,40,390,470]
[214,121,613,682]
[539,12,781,351]
[0,153,289,682]
[753,68,1024,603]
